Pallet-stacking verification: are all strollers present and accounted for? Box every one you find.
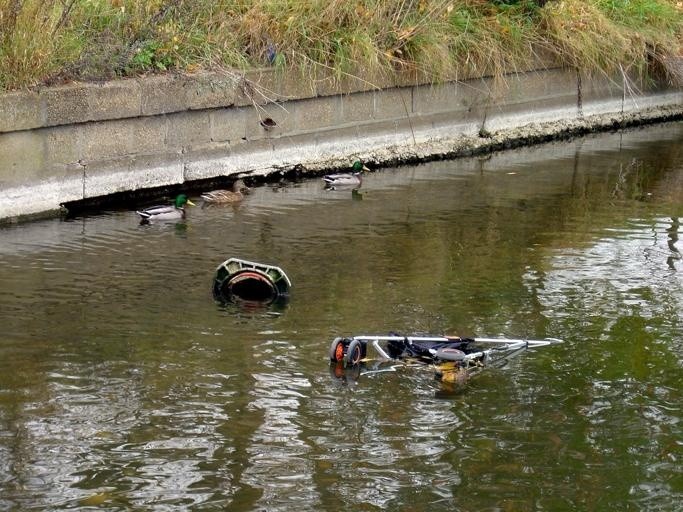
[330,330,565,389]
